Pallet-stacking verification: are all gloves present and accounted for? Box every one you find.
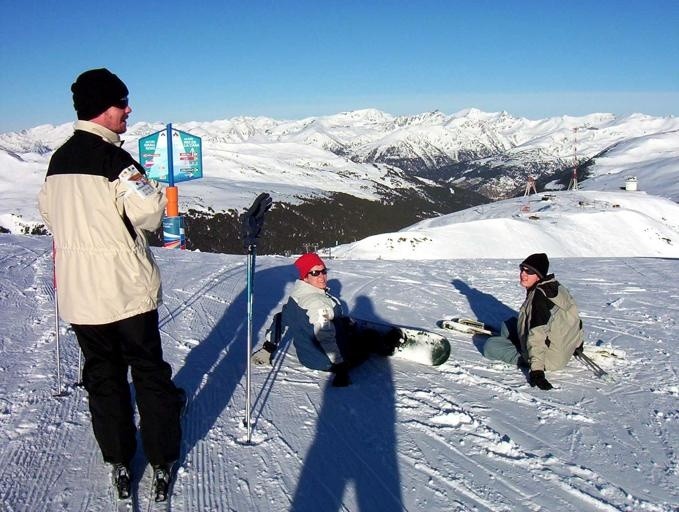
[242,189,275,252]
[529,369,552,391]
[333,371,354,388]
[251,340,276,368]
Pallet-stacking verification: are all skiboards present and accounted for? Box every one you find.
[440,317,626,358]
[113,463,175,512]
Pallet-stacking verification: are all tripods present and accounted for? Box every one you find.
[524,182,537,197]
[566,177,579,192]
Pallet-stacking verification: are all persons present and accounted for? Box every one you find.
[36,65,187,500]
[482,252,586,391]
[251,252,407,387]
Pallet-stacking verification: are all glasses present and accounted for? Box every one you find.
[308,268,327,276]
[520,267,537,275]
[110,98,129,110]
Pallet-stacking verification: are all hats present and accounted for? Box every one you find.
[294,251,325,280]
[71,68,129,120]
[519,253,549,278]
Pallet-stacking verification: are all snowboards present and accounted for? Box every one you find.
[346,315,451,367]
